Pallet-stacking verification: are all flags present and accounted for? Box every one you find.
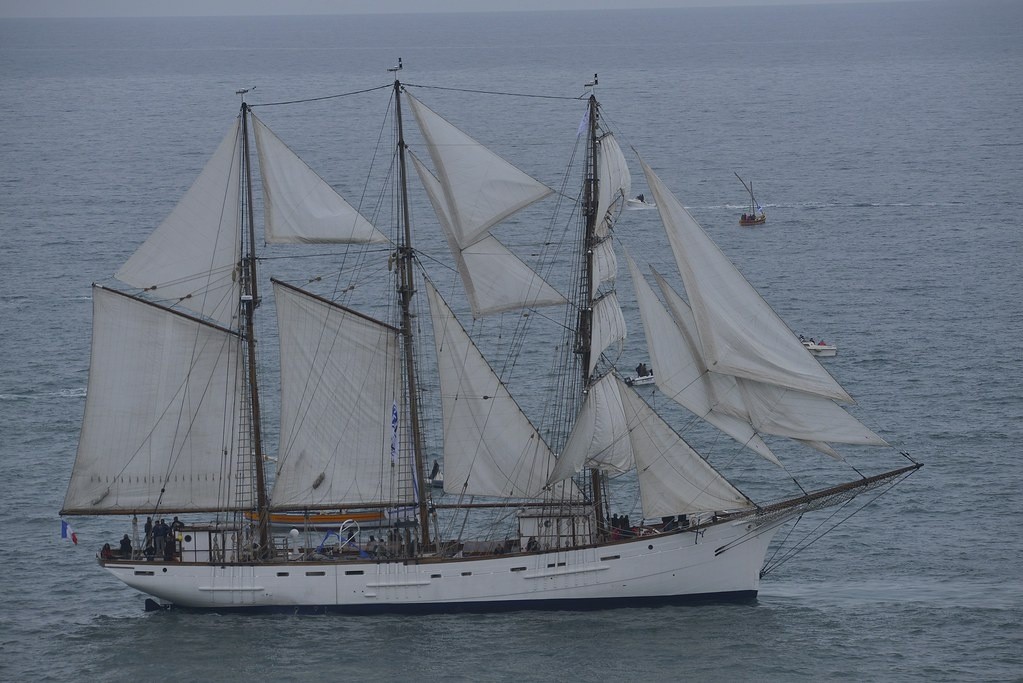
[61,521,77,545]
[389,400,399,463]
[408,437,419,504]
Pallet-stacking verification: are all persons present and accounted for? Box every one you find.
[662,516,674,532]
[347,530,357,542]
[798,334,826,347]
[366,535,377,552]
[144,517,153,549]
[388,529,402,553]
[740,212,756,220]
[678,514,686,526]
[119,533,132,559]
[611,513,629,530]
[526,536,541,553]
[634,363,653,377]
[429,459,440,479]
[373,540,389,555]
[101,543,112,558]
[494,544,504,556]
[153,516,185,556]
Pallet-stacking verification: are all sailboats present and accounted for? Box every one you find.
[733,171,766,226]
[58,56,927,616]
